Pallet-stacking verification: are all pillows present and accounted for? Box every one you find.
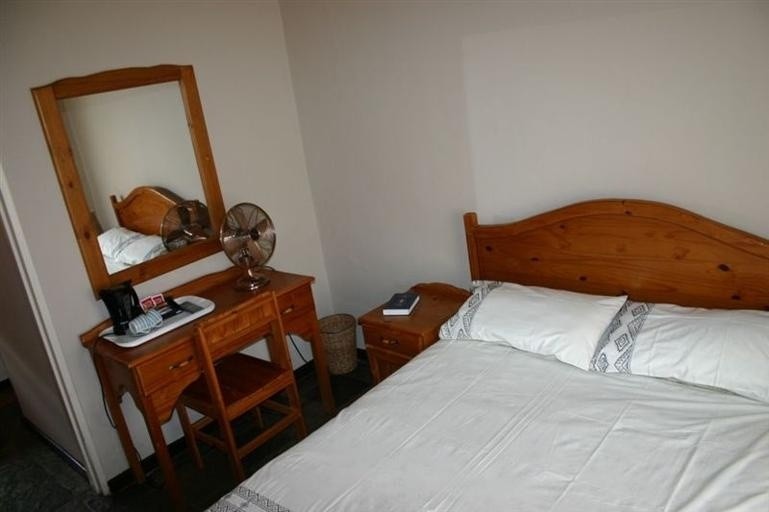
[438,279,629,372]
[590,299,769,405]
[97,227,166,266]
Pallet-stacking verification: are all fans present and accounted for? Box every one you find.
[160,200,213,251]
[218,202,277,292]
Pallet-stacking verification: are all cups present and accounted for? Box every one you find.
[128,308,164,336]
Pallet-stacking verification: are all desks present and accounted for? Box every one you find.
[80,265,337,512]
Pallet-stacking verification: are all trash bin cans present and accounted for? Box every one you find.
[318,313,357,376]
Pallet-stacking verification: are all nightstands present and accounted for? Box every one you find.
[358,282,472,384]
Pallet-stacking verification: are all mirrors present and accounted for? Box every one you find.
[30,64,230,301]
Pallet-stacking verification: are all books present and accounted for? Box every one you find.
[382,293,421,316]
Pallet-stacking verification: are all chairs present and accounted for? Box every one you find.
[176,289,307,486]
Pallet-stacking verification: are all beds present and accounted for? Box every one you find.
[96,186,203,277]
[206,198,769,512]
